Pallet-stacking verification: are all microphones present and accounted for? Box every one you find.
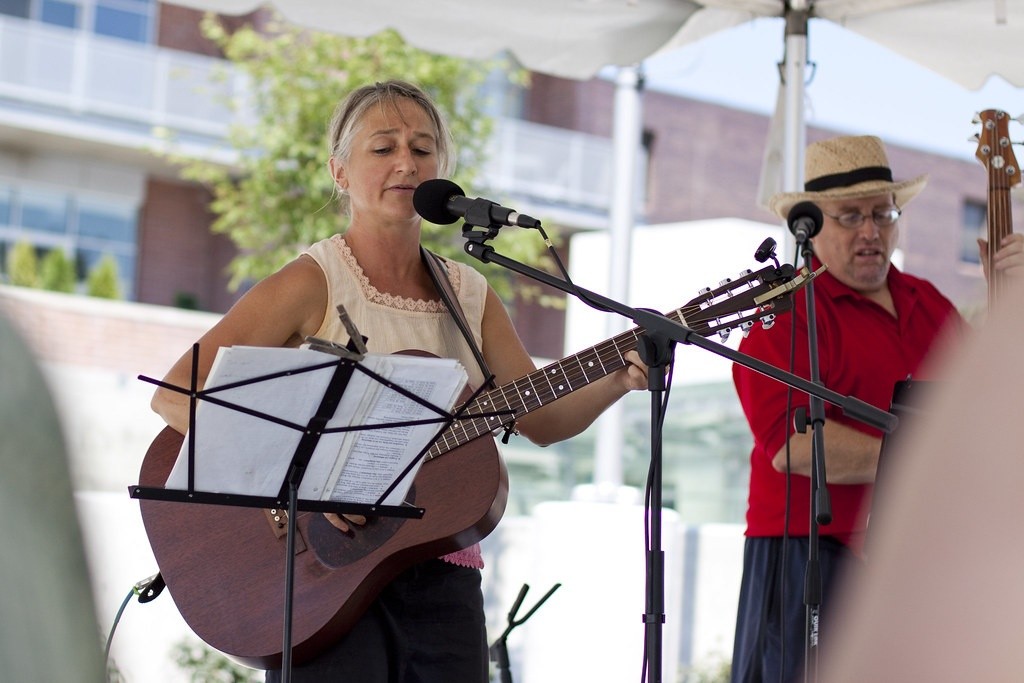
[412,179,541,229]
[786,202,824,243]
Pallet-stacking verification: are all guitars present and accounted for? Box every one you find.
[136,258,829,673]
[965,103,1024,314]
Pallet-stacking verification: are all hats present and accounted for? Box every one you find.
[770,136,930,224]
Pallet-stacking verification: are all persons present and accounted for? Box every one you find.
[731,135,1024,683]
[150,82,650,683]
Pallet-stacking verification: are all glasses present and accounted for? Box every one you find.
[824,207,902,228]
